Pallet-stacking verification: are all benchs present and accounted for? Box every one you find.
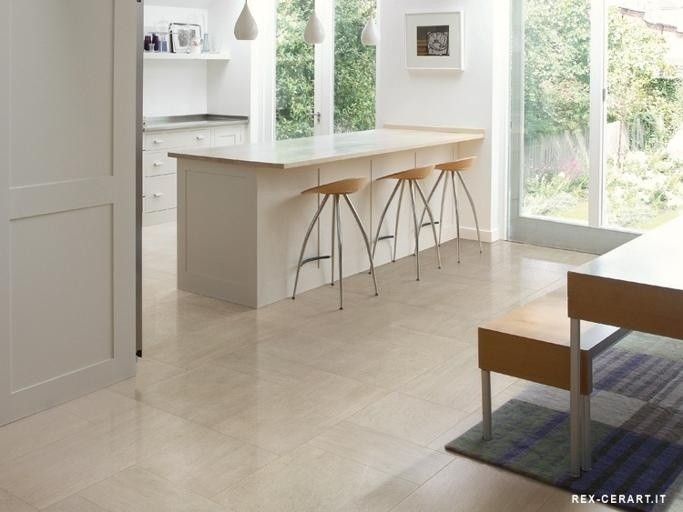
[478,286,631,472]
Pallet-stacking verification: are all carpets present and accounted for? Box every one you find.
[445,328,683,511]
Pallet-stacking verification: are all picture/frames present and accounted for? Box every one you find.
[403,9,465,72]
[169,22,201,53]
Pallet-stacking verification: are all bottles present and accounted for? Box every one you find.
[201,33,211,53]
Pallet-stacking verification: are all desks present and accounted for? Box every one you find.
[567,213,683,478]
[168,123,485,310]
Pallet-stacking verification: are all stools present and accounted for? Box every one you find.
[412,156,483,263]
[291,176,378,309]
[368,164,441,281]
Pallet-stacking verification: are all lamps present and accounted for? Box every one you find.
[361,1,378,46]
[304,0,324,43]
[234,0,259,41]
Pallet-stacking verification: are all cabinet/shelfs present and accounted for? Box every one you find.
[141,53,249,223]
